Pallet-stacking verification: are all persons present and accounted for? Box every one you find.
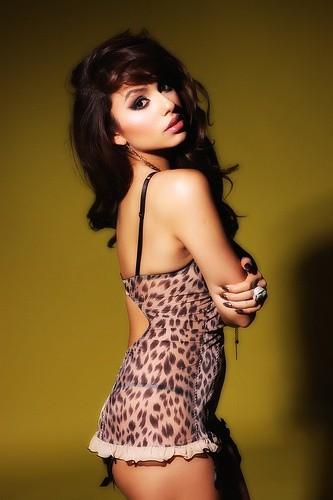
[68,29,267,500]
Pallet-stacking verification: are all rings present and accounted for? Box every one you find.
[253,286,267,304]
